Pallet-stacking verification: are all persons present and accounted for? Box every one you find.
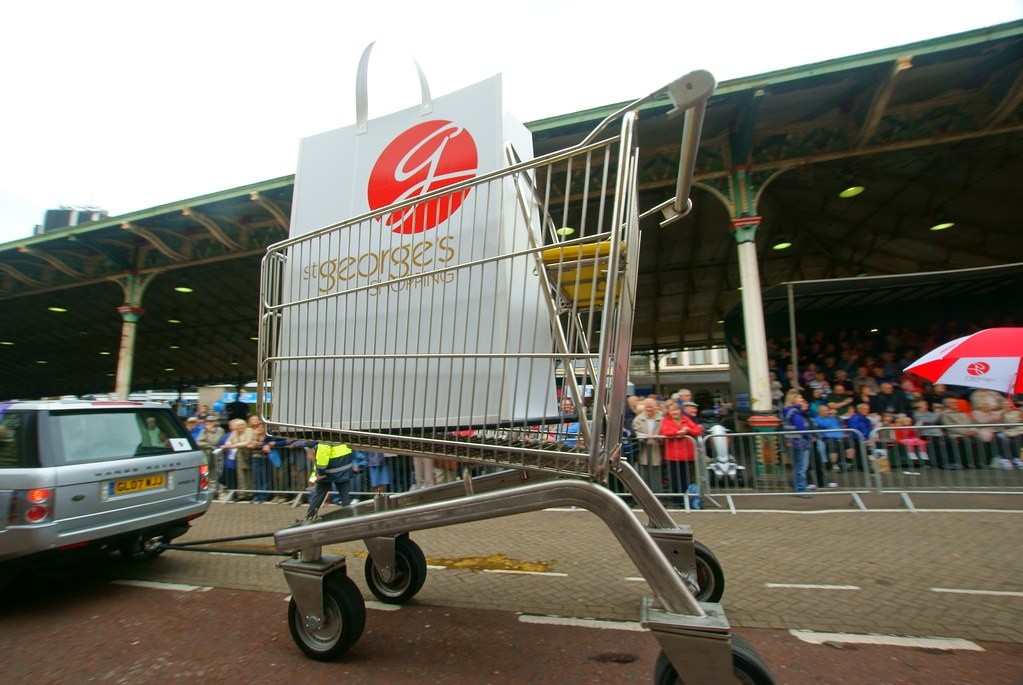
[185,416,205,442]
[795,398,832,490]
[632,399,665,502]
[553,383,642,497]
[142,417,172,448]
[218,416,552,503]
[308,442,354,520]
[663,400,704,510]
[197,416,225,502]
[779,387,817,499]
[636,326,1023,470]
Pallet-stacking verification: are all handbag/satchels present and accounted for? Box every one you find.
[781,408,808,439]
[270,39,558,433]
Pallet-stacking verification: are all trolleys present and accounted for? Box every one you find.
[255,68,775,685]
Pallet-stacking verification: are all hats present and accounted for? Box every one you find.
[683,401,699,406]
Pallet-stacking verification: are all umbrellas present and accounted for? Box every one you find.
[902,326,1023,397]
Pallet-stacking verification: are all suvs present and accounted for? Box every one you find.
[0,399,214,596]
